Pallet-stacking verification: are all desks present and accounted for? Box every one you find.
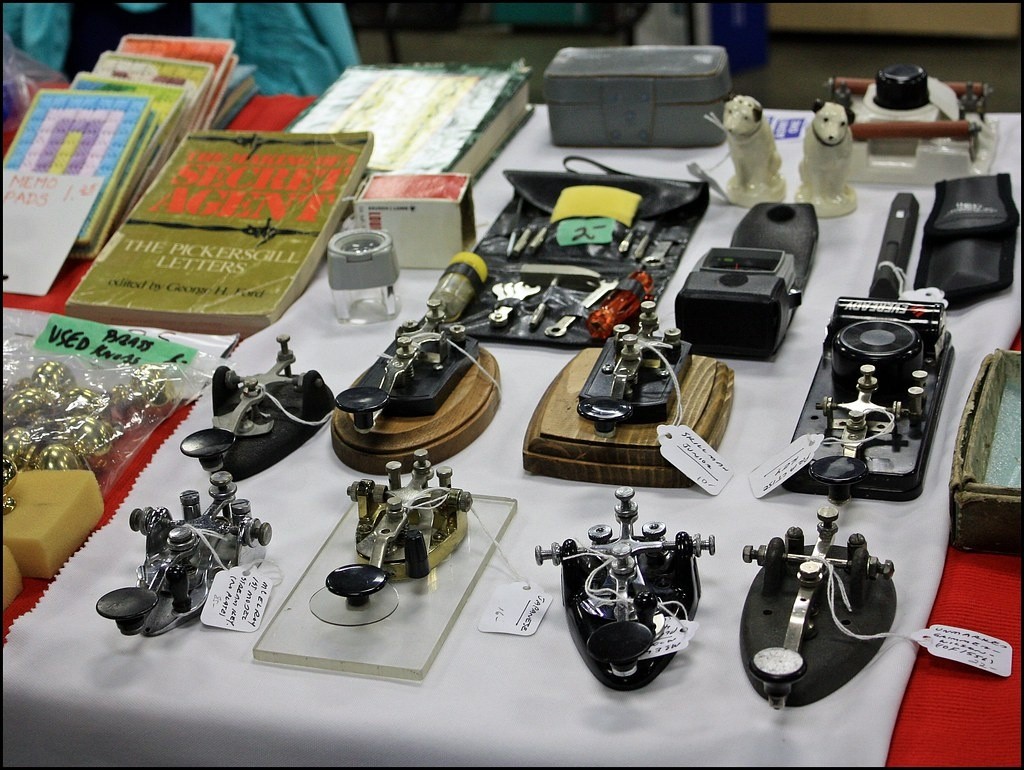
[2,78,1023,768]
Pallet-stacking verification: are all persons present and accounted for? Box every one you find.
[1,0,358,116]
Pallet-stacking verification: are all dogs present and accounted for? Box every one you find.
[722,95,787,208]
[795,99,858,218]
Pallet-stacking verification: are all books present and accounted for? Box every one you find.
[2,34,373,328]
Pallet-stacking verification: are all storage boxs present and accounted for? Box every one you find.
[950,348,1023,557]
